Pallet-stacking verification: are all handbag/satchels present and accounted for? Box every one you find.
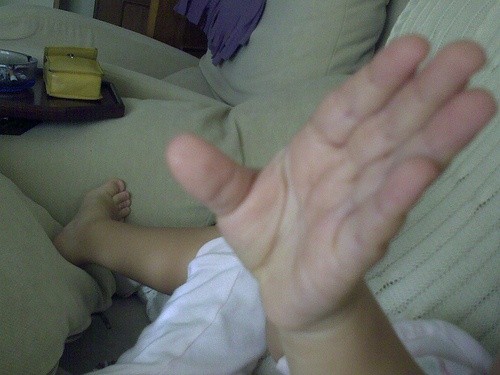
[42,44,104,100]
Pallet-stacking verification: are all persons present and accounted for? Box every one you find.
[165,32,498,375]
[52,179,493,375]
